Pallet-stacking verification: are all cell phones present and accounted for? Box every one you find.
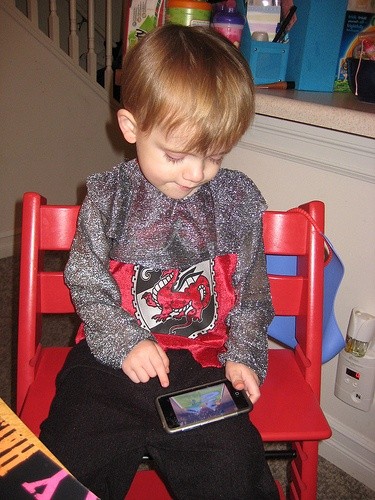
[155,379,254,435]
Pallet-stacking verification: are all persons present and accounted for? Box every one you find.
[37,23,281,499]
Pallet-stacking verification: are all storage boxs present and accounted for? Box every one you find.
[235,0,289,87]
[122,0,166,58]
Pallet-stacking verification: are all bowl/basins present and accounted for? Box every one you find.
[346,57,375,103]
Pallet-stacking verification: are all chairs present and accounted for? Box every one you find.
[17,192,332,500]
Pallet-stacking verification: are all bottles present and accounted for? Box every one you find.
[210,0,245,50]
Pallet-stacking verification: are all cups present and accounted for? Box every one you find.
[165,0,213,29]
